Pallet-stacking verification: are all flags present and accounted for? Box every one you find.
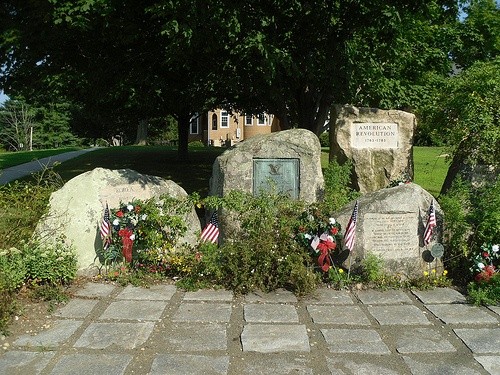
[344,199,358,251]
[200,208,220,247]
[98,201,112,250]
[423,200,436,246]
